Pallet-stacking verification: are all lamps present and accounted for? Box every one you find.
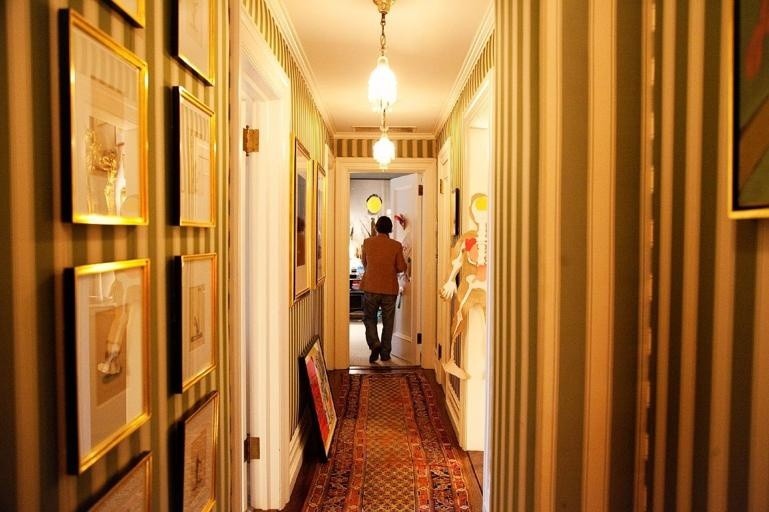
[365,1,399,166]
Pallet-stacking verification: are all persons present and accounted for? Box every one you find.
[358,216,408,362]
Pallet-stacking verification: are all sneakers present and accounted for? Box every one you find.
[370,341,383,362]
[381,352,390,361]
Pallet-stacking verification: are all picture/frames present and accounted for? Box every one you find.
[451,188,459,236]
[289,135,327,309]
[297,334,338,462]
